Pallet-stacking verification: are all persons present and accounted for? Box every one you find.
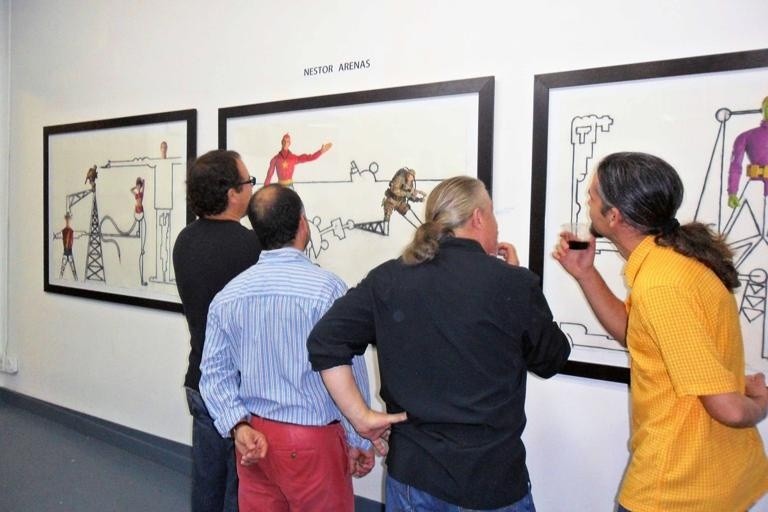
[200,184,374,512]
[60,211,75,257]
[130,177,146,214]
[85,165,99,190]
[552,151,767,512]
[161,142,168,158]
[307,177,570,511]
[726,97,767,207]
[173,150,263,512]
[379,167,425,223]
[264,134,332,191]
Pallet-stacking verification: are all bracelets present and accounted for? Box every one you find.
[230,422,251,442]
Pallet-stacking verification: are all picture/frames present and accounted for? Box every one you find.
[42,109,198,313]
[528,48,768,383]
[217,75,496,287]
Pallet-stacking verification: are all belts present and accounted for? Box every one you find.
[250,412,340,426]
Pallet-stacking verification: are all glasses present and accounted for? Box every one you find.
[240,176,256,185]
